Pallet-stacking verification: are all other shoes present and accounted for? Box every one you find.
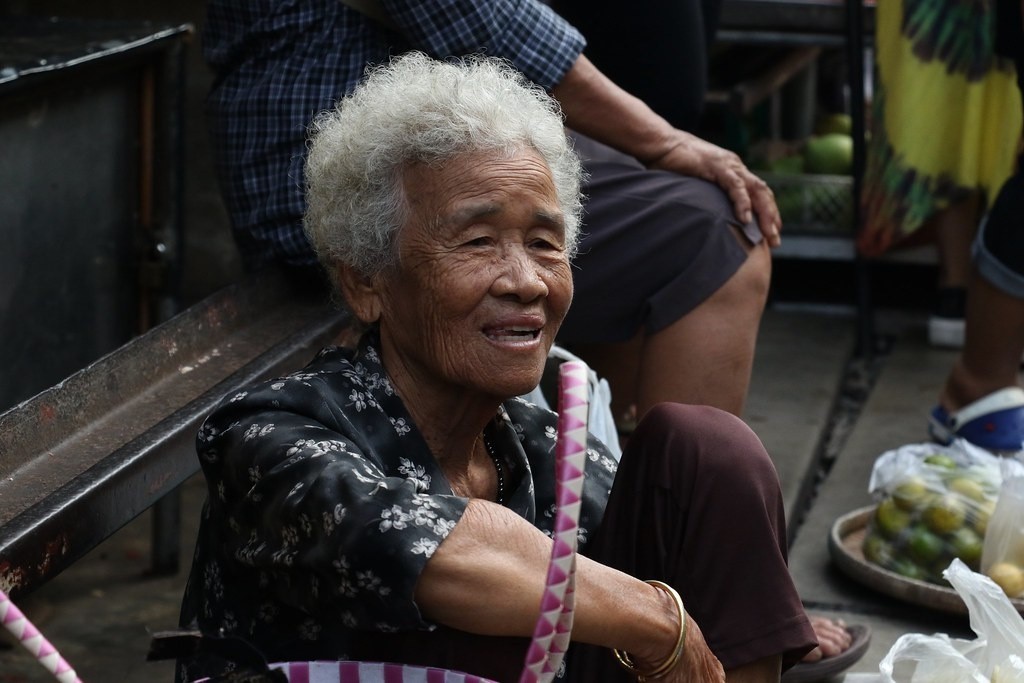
[926,285,968,345]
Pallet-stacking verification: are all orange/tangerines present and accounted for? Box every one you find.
[864,455,1024,601]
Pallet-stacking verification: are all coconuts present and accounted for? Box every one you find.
[753,112,853,228]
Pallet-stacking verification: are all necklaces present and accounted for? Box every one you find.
[484,432,504,505]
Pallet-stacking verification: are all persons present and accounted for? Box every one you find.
[179,53,871,683]
[854,0,1024,452]
[202,0,782,416]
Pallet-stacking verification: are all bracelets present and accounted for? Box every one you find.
[612,579,686,683]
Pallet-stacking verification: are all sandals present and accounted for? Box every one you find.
[780,621,873,683]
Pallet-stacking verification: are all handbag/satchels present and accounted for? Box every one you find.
[867,435,1024,683]
